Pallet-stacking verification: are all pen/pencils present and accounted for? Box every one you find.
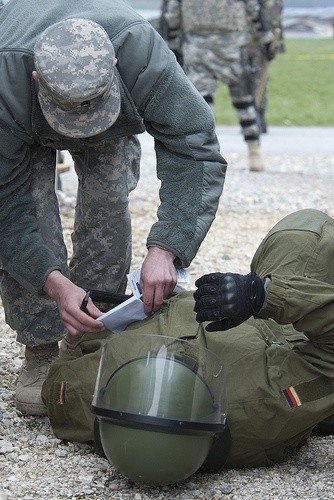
[78,291,92,310]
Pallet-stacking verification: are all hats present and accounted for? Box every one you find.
[33,18,121,138]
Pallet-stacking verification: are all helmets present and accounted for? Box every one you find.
[97,356,217,486]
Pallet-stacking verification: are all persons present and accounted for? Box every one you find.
[161,0,285,171]
[42,209,334,486]
[0,0,227,417]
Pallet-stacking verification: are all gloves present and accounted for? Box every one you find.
[193,272,266,333]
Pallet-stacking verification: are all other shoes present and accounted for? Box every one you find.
[15,340,61,415]
[249,143,264,171]
